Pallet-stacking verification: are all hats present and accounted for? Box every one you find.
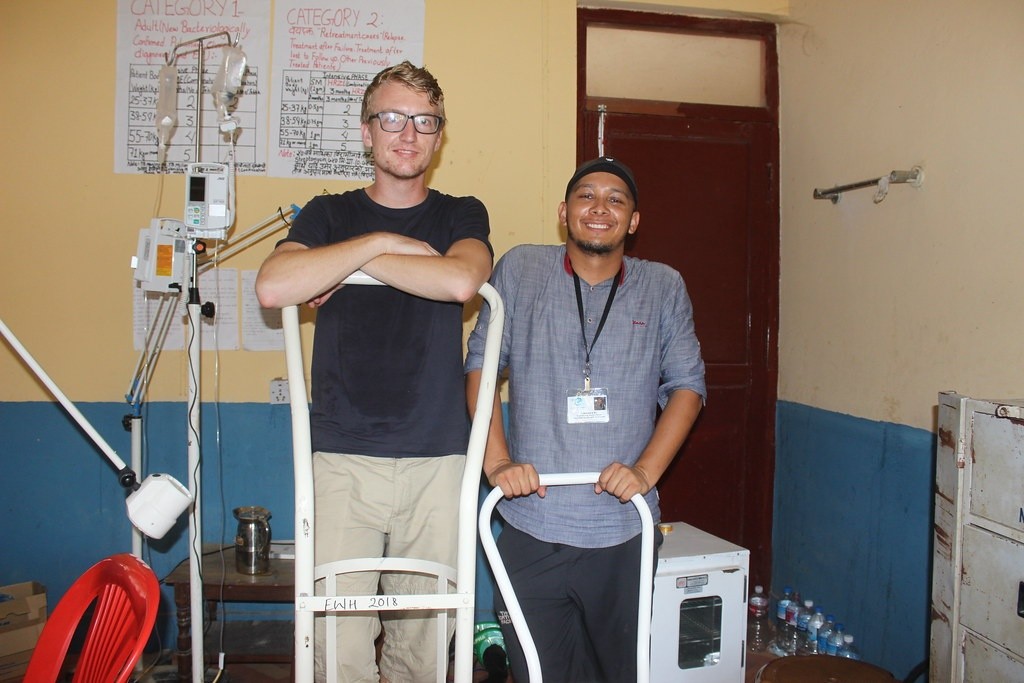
[564,156,638,209]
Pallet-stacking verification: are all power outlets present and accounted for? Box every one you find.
[270,379,290,405]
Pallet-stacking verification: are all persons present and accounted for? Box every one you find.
[255,59,494,683]
[461,156,708,682]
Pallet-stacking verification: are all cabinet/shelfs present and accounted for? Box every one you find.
[650,522,750,683]
[928,390,1024,683]
[163,544,295,683]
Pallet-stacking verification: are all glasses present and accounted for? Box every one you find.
[368,111,442,134]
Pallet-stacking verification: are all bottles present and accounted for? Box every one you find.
[748,586,769,649]
[777,586,859,659]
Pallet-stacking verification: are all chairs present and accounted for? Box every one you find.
[22,553,160,683]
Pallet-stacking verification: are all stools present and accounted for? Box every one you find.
[754,654,898,683]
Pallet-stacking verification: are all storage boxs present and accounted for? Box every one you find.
[0,580,48,683]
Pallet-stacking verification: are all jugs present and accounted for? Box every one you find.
[233,506,271,575]
